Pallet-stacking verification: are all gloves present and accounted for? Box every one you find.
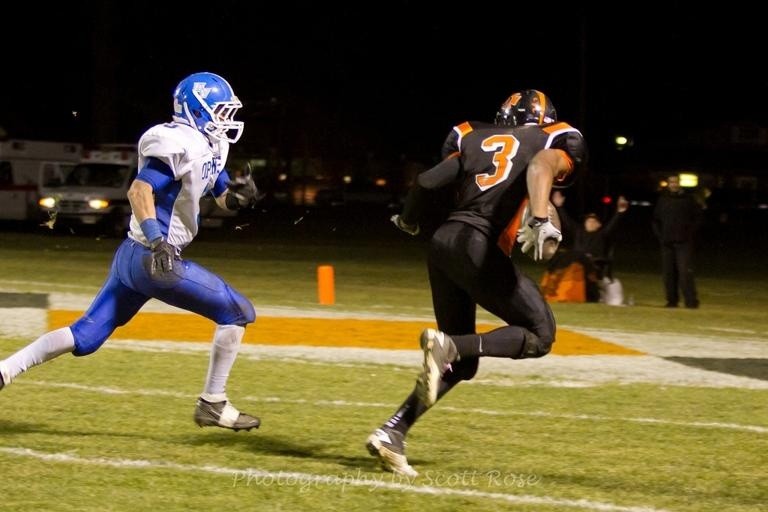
[148,236,183,276]
[516,216,563,262]
[390,215,420,236]
[224,162,259,210]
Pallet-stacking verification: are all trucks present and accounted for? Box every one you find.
[35,141,140,233]
[0,137,86,224]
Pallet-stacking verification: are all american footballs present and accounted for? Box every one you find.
[520,201,561,264]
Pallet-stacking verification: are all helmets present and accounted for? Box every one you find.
[173,72,244,143]
[494,89,557,127]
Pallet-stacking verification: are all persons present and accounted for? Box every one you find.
[0,70,262,432]
[365,89,588,476]
[551,189,630,303]
[653,174,702,308]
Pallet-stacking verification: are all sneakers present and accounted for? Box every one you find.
[365,428,419,477]
[416,328,458,407]
[193,398,260,433]
